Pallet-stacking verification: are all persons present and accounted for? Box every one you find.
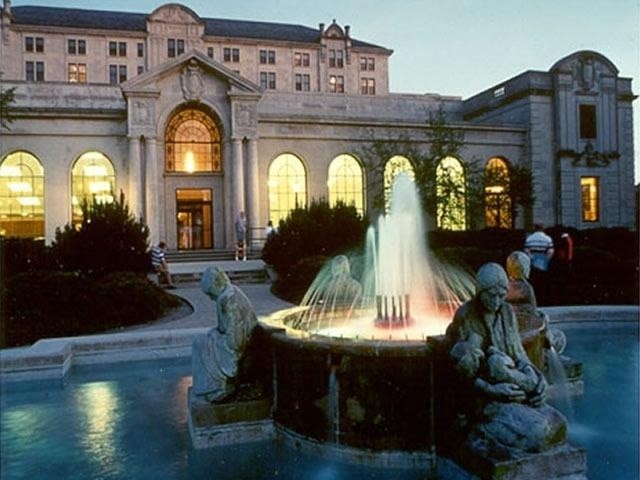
[151,240,178,289]
[233,239,247,262]
[505,250,567,356]
[189,266,259,401]
[446,257,568,456]
[264,220,273,235]
[525,222,555,269]
[557,231,574,259]
[327,255,364,296]
[234,211,247,239]
[450,336,538,402]
[275,219,285,234]
